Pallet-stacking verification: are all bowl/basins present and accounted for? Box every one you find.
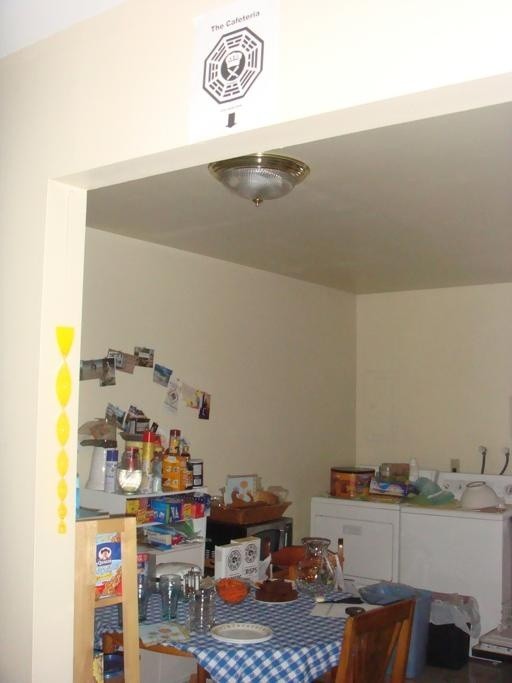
[104,654,125,683]
[459,480,501,509]
[217,578,249,604]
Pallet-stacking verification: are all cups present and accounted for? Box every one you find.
[137,574,151,623]
[187,589,216,637]
[159,573,181,622]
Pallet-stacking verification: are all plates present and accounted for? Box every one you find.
[210,622,274,643]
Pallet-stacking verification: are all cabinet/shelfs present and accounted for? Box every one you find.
[76,515,140,683]
[80,487,208,577]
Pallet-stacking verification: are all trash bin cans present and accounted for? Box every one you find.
[427,592,474,669]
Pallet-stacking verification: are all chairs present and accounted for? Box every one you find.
[264,536,344,583]
[312,597,416,683]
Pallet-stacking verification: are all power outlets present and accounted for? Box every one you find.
[450,458,460,472]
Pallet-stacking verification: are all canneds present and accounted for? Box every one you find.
[369,480,408,497]
[187,459,203,489]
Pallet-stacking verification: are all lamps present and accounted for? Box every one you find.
[208,152,310,207]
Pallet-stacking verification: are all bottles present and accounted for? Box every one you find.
[295,537,338,598]
[103,426,193,493]
[408,458,419,482]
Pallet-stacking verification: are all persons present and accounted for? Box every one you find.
[100,547,111,561]
